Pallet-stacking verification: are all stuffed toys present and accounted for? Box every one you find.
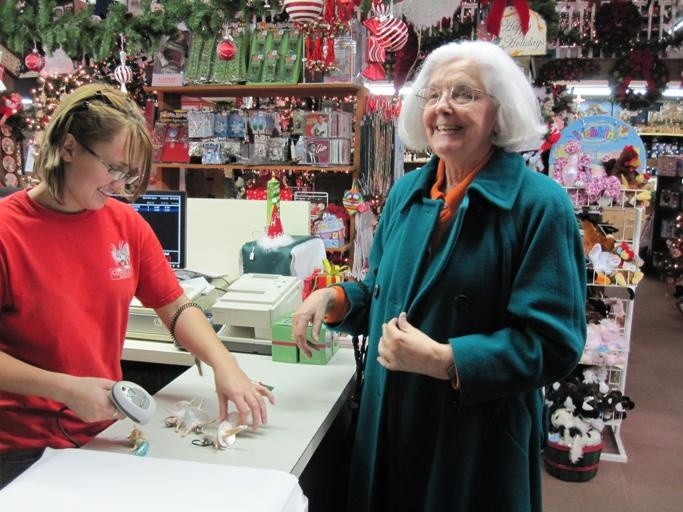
[546,144,655,464]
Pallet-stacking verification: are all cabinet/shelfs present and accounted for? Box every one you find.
[634,127,682,282]
[542,186,650,467]
[142,82,369,274]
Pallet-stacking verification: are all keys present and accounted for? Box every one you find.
[194,357,203,377]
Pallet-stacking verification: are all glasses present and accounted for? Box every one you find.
[413,79,498,109]
[72,131,141,185]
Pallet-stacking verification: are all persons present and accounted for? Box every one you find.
[0,78,274,488]
[309,114,328,138]
[292,41,587,511]
[307,143,319,163]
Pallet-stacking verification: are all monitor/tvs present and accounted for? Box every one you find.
[109,190,187,269]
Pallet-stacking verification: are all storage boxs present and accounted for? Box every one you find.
[270,315,340,368]
